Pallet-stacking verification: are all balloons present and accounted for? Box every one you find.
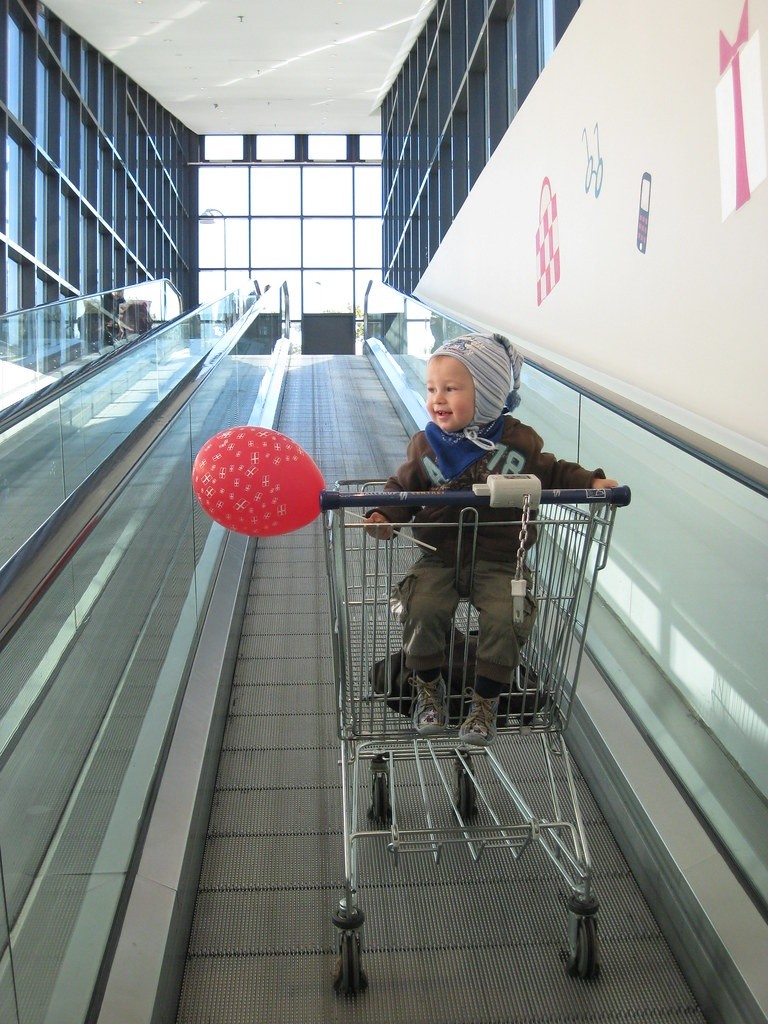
[192,426,327,536]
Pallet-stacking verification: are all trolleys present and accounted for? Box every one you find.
[319,471,631,1000]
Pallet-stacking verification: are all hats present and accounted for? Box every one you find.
[427,332,524,451]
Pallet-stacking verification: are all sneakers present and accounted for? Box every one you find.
[458,686,500,746]
[408,672,450,734]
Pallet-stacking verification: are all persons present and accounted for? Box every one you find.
[360,333,619,745]
[103,291,126,346]
